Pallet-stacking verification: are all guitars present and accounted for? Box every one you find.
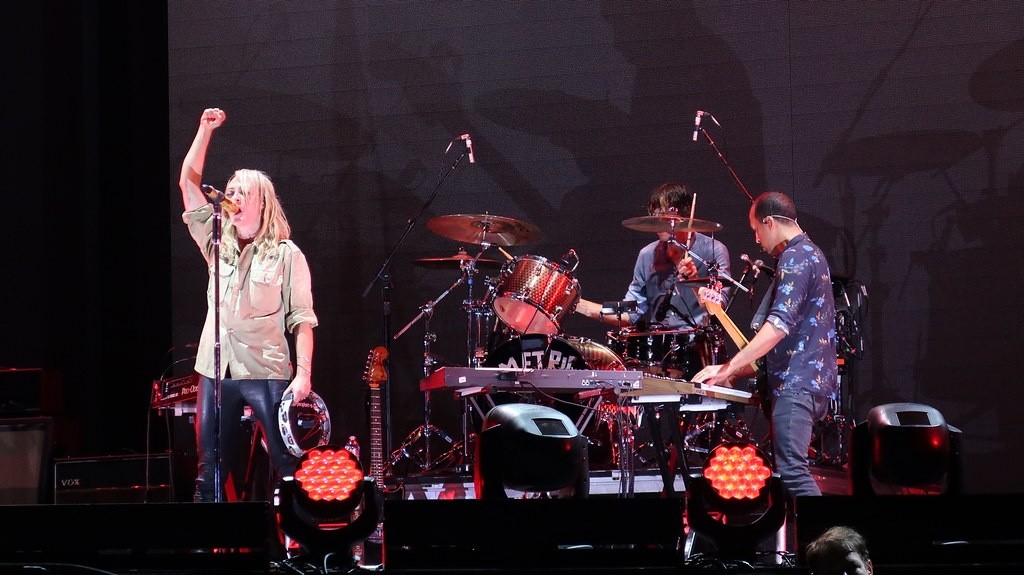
[698,286,772,422]
[349,345,405,572]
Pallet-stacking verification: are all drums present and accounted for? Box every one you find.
[471,333,640,470]
[608,329,690,379]
[490,254,582,335]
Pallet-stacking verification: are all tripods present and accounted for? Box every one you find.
[383,235,491,476]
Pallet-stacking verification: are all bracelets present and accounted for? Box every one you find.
[297,355,310,363]
[296,363,311,373]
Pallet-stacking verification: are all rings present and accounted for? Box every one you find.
[303,398,306,399]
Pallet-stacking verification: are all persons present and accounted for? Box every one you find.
[573,182,735,406]
[690,190,839,497]
[806,526,874,575]
[177,107,320,513]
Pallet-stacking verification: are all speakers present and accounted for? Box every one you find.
[0,504,284,566]
[793,495,1024,566]
[0,417,55,504]
[382,492,686,568]
[54,457,173,504]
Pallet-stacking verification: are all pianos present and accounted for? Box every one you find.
[419,364,644,473]
[150,361,295,501]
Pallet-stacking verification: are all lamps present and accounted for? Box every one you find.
[687,440,786,556]
[279,444,379,555]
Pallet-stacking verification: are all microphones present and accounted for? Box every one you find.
[568,250,573,259]
[753,260,775,276]
[656,285,675,322]
[602,300,639,315]
[454,133,475,163]
[201,184,239,215]
[741,254,760,274]
[693,110,709,140]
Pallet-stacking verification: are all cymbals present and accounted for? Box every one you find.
[622,215,725,233]
[277,389,332,458]
[414,254,503,269]
[426,213,547,247]
[676,275,748,289]
